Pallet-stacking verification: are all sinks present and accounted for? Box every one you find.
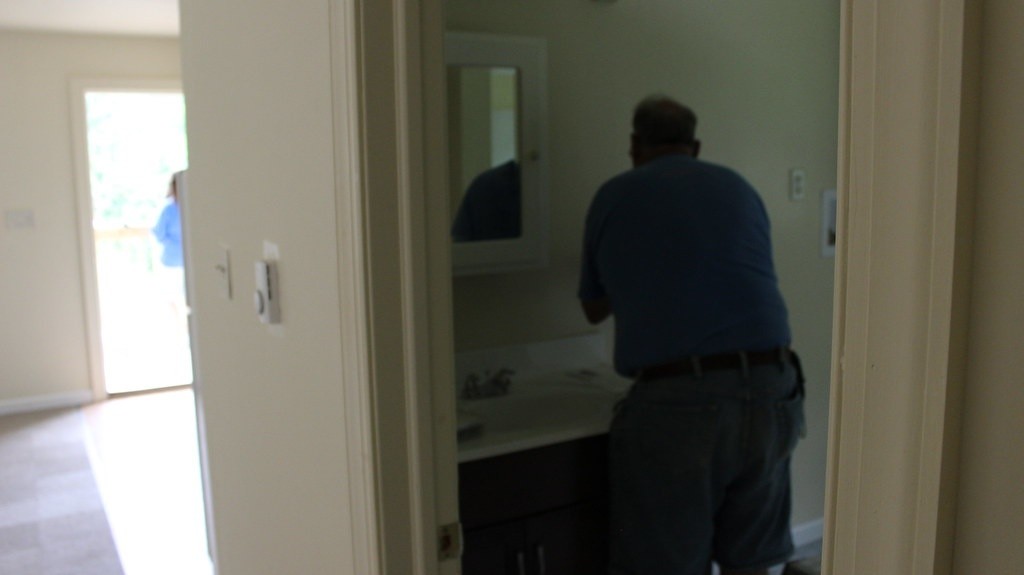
[455,382,634,464]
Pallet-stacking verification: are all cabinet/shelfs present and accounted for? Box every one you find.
[459,432,614,575]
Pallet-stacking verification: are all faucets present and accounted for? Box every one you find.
[461,367,516,401]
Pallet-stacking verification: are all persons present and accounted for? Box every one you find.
[451,161,522,242]
[575,96,807,575]
[153,171,183,269]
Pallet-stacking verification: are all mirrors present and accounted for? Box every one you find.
[443,31,550,277]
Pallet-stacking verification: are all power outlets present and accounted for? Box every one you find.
[791,169,805,201]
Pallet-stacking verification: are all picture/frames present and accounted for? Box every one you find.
[821,189,835,255]
